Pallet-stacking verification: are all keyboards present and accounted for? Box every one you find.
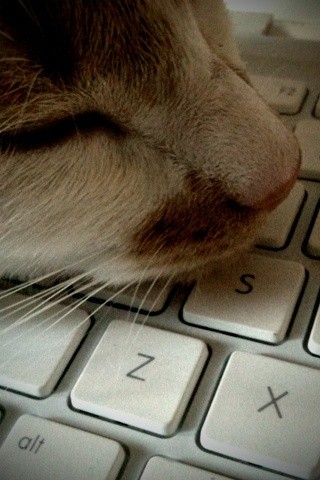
[0,10,320,480]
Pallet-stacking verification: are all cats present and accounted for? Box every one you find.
[0,0,301,368]
